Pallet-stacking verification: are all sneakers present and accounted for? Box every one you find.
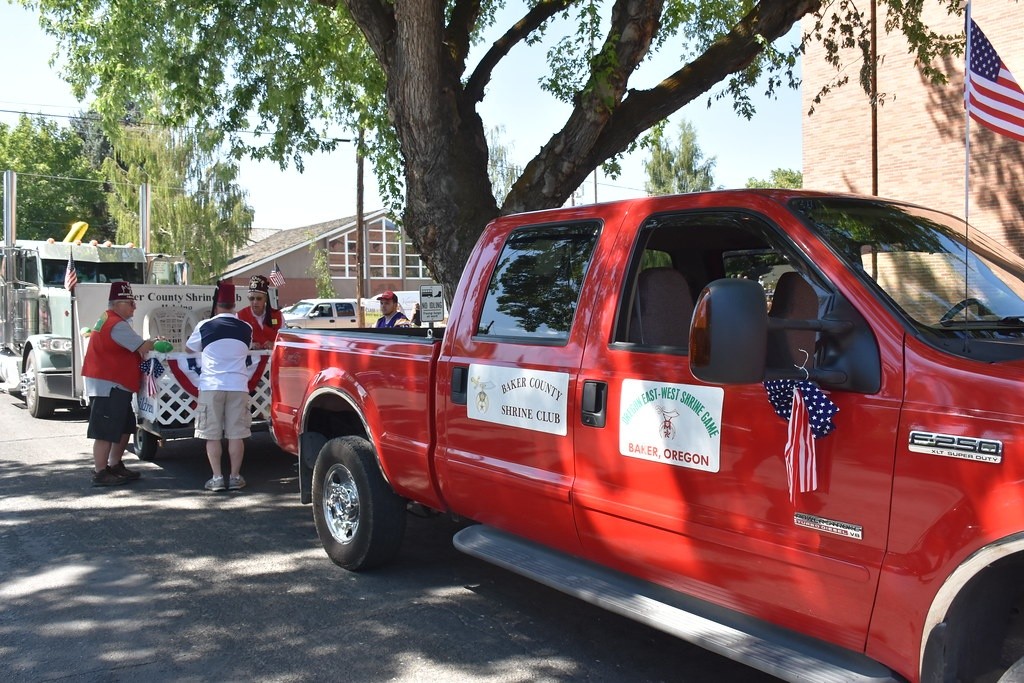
[205,475,226,490]
[228,474,246,489]
[106,460,141,479]
[90,465,129,485]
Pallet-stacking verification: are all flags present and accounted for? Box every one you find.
[270,264,286,286]
[64,254,78,291]
[964,11,1024,143]
[141,357,164,398]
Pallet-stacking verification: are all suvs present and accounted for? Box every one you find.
[279,298,358,329]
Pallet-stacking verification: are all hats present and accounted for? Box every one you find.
[249,275,273,326]
[376,291,398,303]
[109,282,135,299]
[210,280,235,318]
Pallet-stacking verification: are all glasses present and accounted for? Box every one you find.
[118,300,136,306]
[247,296,264,301]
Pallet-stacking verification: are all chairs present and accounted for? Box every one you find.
[624,266,694,347]
[765,272,820,368]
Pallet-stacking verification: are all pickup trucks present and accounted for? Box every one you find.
[269,188,1024,683]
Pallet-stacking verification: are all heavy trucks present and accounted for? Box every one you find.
[0,170,191,418]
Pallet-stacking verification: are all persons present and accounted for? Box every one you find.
[411,303,421,328]
[185,284,253,491]
[235,274,285,350]
[376,291,411,328]
[80,281,165,485]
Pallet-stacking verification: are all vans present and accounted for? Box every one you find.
[365,290,448,318]
